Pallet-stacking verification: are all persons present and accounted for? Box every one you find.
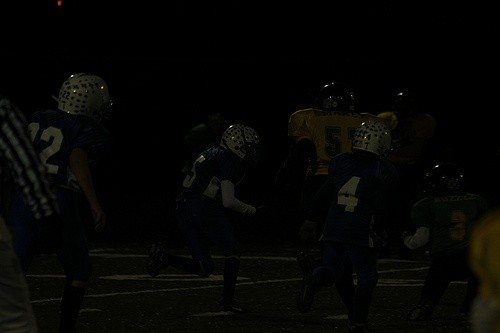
[288,80,381,313]
[146,123,264,314]
[316,119,406,332]
[377,92,437,259]
[407,157,488,320]
[0,98,59,333]
[469,206,500,333]
[183,104,232,171]
[5,73,114,333]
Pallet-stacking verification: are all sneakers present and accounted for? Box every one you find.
[296,250,325,312]
[219,300,249,313]
[147,243,163,276]
[409,299,433,320]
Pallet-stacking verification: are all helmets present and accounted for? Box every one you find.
[219,124,259,159]
[57,73,113,120]
[316,82,355,112]
[352,120,391,159]
[423,161,464,193]
